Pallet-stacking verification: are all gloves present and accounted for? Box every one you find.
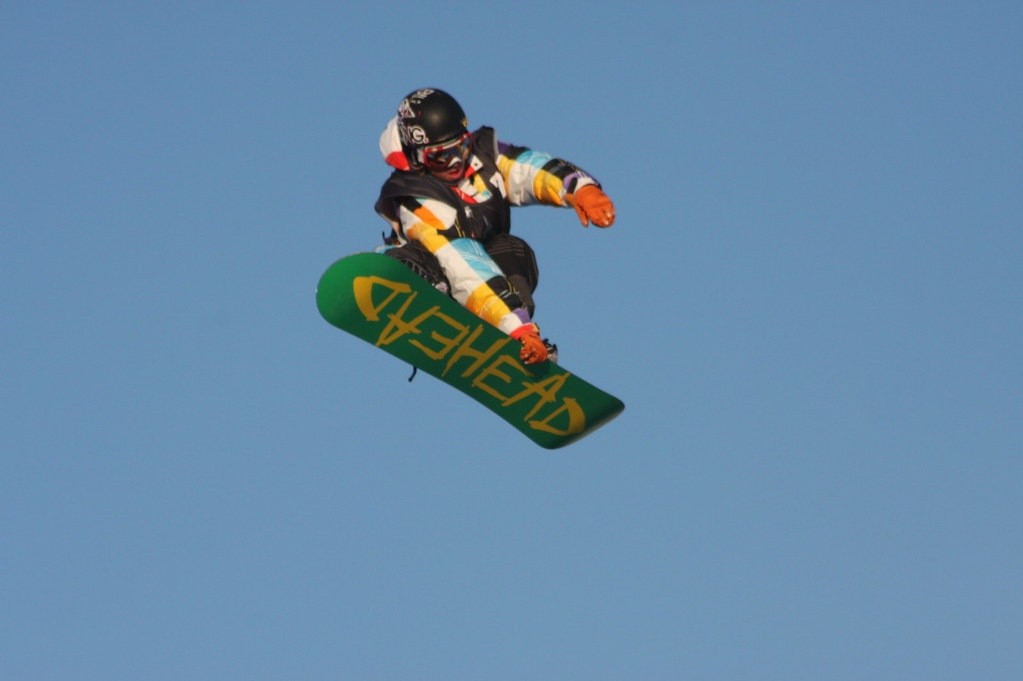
[511,322,549,366]
[567,184,615,227]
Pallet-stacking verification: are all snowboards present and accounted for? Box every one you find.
[315,252,625,450]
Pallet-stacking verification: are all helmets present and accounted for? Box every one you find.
[396,87,465,163]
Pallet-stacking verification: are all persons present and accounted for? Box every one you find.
[374,87,616,365]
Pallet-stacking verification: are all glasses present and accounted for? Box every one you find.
[425,133,474,170]
